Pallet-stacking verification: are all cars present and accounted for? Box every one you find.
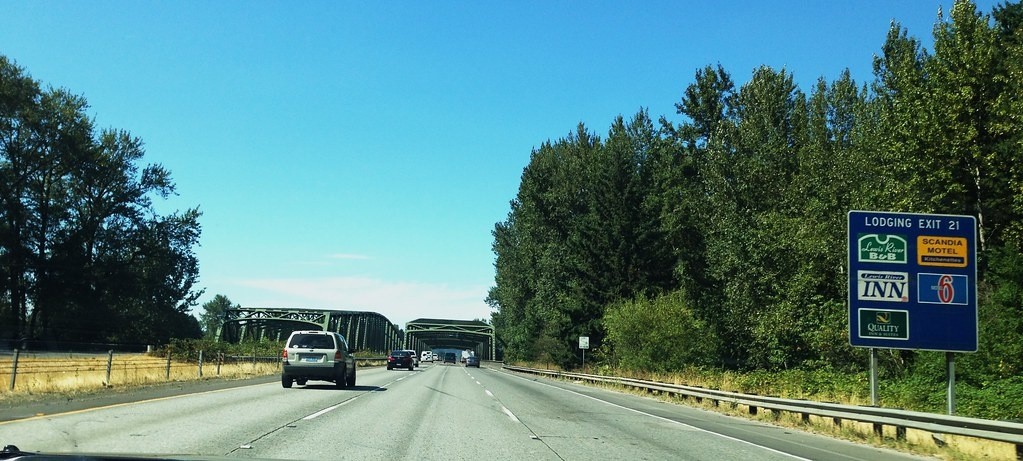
[466,356,481,368]
[387,351,416,371]
[404,350,420,367]
[433,354,440,361]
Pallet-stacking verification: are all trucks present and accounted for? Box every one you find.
[461,351,475,361]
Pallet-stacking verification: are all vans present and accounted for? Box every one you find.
[421,350,434,363]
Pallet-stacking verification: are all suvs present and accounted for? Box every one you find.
[280,331,360,389]
[444,353,459,364]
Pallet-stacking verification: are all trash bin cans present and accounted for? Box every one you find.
[147,343,154,352]
